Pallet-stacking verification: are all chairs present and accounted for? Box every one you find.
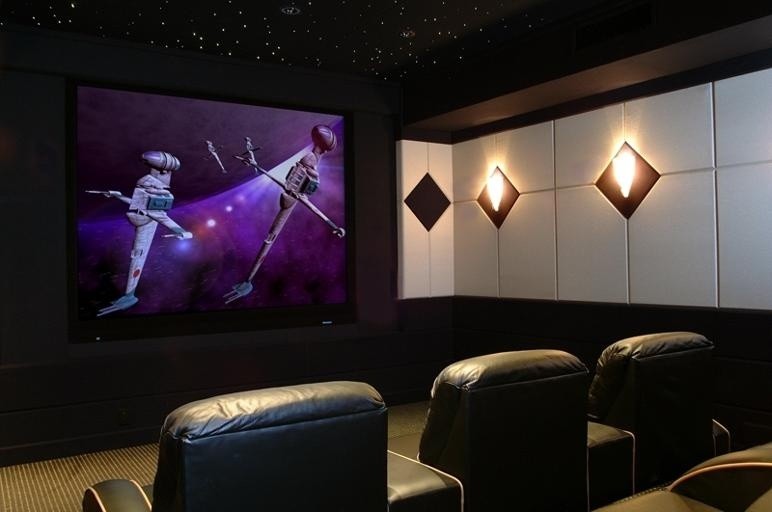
[418,350,635,511]
[588,441,770,512]
[586,330,731,510]
[83,379,465,511]
